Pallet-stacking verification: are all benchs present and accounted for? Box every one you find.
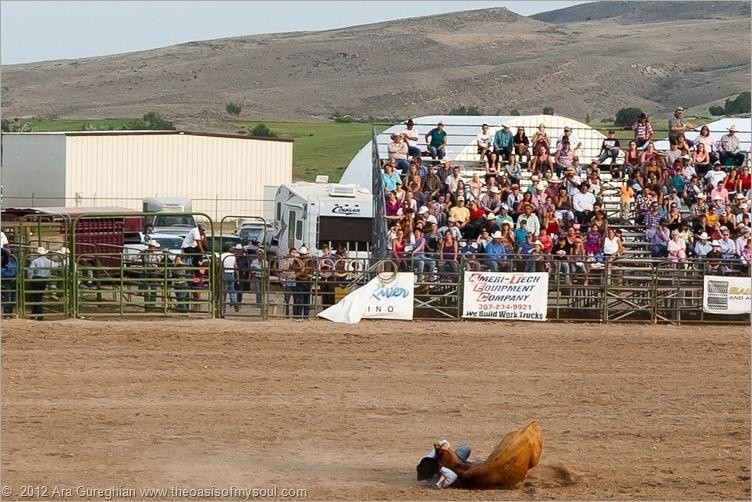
[383,161,656,261]
[520,260,522,262]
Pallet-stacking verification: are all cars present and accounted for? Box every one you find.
[123,197,278,273]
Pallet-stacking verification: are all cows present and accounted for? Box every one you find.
[432,420,542,490]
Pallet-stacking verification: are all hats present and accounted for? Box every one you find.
[199,223,207,230]
[36,246,71,255]
[233,243,243,250]
[385,118,751,248]
[146,240,161,248]
[299,243,343,254]
[256,249,264,253]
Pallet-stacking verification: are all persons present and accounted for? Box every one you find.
[132,220,347,320]
[417,441,471,489]
[1,232,76,320]
